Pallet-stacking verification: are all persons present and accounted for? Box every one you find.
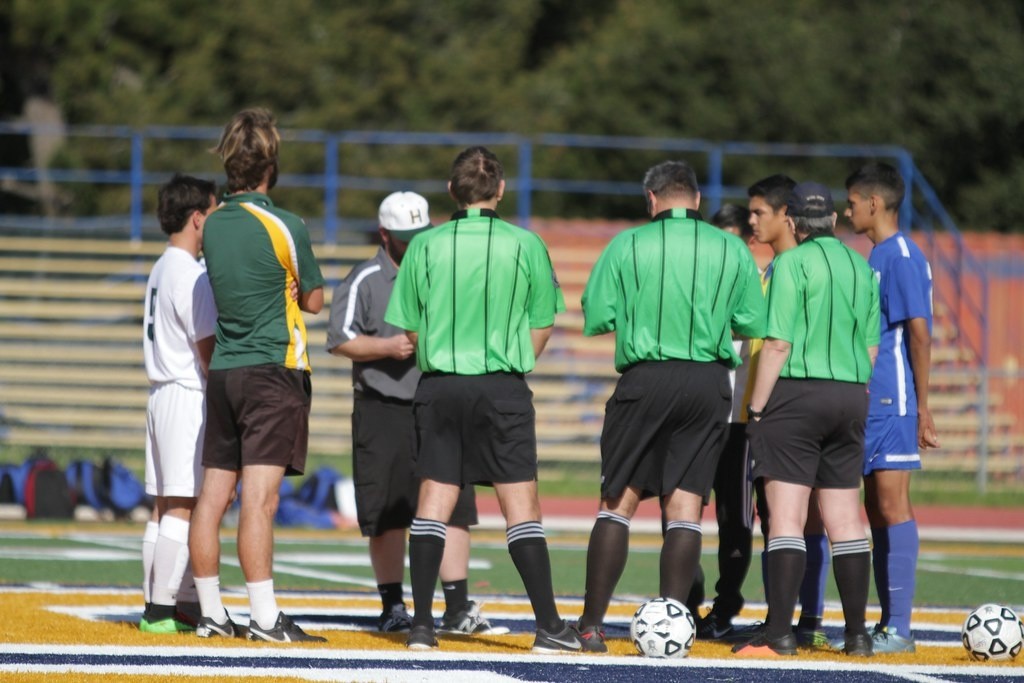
[191,109,328,641]
[140,175,219,632]
[683,174,829,647]
[731,185,882,655]
[384,144,581,653]
[833,160,941,653]
[567,160,767,653]
[325,190,509,636]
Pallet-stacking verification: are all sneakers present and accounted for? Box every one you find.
[531,620,582,654]
[246,611,328,644]
[435,600,511,637]
[696,611,734,641]
[829,626,875,651]
[570,617,607,654]
[870,623,916,654]
[377,611,414,635]
[196,607,251,640]
[403,623,440,651]
[730,623,797,654]
[139,614,197,635]
[843,630,873,656]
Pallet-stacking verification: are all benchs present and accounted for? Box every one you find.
[0,235,1023,473]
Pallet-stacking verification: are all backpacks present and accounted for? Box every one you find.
[1,445,343,535]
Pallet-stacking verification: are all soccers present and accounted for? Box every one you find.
[960,603,1024,663]
[629,596,698,659]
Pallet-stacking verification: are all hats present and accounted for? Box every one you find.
[785,184,836,218]
[379,191,435,241]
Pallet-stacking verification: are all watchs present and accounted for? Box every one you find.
[747,404,767,416]
